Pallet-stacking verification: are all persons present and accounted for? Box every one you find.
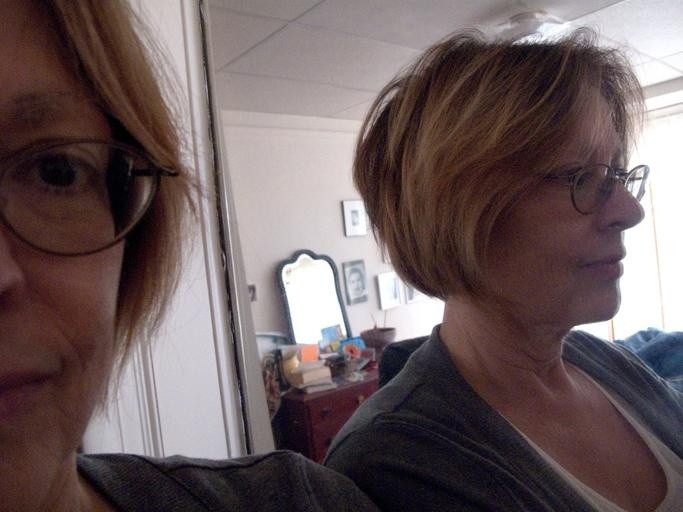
[322,21,683,510]
[346,267,366,297]
[0,1,375,512]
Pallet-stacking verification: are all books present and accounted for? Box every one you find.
[290,366,338,394]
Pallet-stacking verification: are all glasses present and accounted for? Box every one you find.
[1,136,180,259]
[546,163,652,218]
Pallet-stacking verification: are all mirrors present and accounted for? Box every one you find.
[274,249,353,349]
[185,1,683,510]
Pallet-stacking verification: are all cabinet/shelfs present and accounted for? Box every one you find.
[281,368,379,462]
